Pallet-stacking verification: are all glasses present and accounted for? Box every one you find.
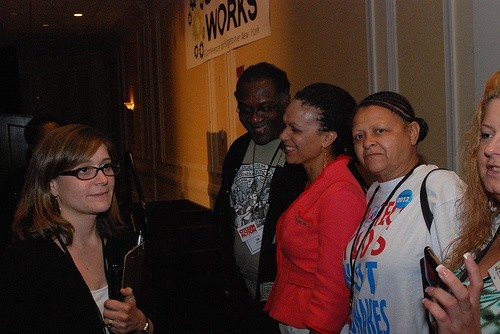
[424,245,467,329]
[236,96,286,118]
[59,163,122,180]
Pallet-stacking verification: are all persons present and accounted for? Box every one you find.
[343,91,472,334]
[263,82,370,334]
[212,63,309,334]
[422,70,500,334]
[0,124,153,334]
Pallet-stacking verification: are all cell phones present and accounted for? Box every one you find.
[424,246,452,294]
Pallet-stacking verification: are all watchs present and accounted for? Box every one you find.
[141,316,150,334]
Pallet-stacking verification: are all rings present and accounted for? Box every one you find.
[108,319,112,329]
